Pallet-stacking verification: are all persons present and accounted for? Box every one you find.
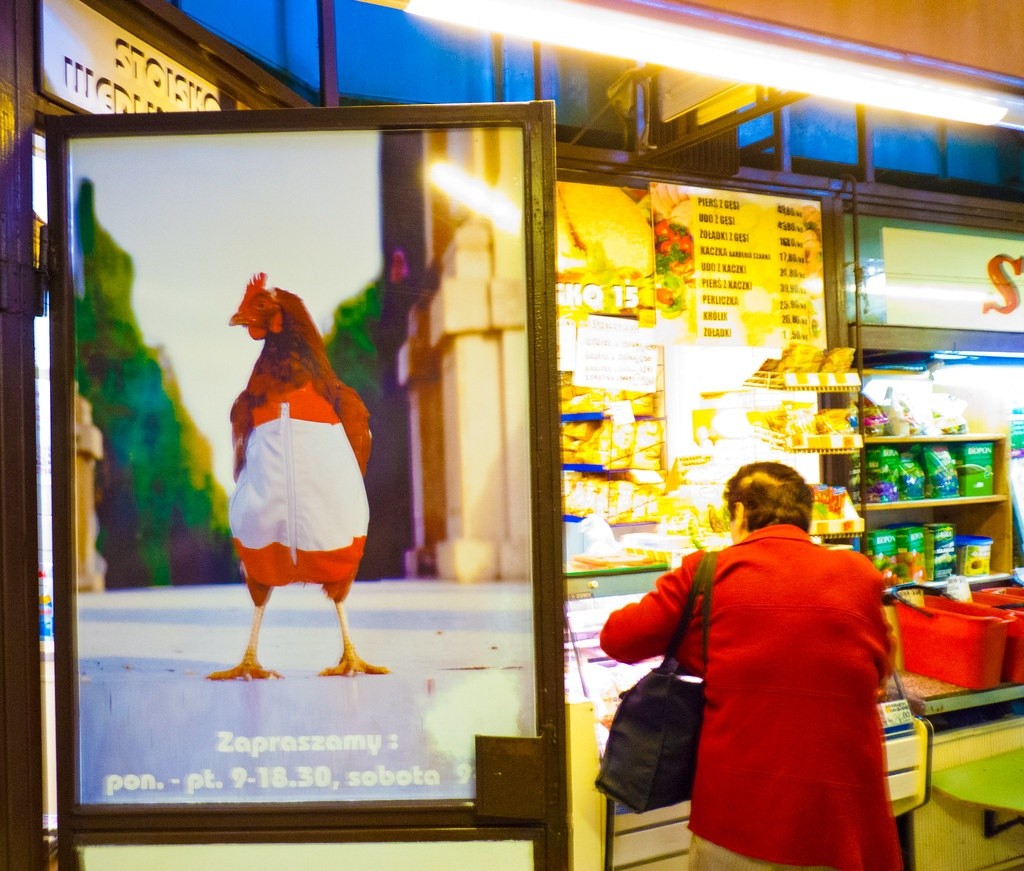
[600,462,903,871]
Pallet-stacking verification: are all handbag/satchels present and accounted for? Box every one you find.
[594,551,716,814]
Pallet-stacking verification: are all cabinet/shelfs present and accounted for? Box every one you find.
[827,429,1015,601]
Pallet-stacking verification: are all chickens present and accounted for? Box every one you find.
[204,270,389,682]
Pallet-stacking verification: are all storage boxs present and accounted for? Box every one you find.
[971,586,1024,686]
[892,584,1018,690]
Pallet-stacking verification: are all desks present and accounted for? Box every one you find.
[931,745,1024,841]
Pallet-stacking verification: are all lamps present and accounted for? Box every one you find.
[404,0,1008,127]
